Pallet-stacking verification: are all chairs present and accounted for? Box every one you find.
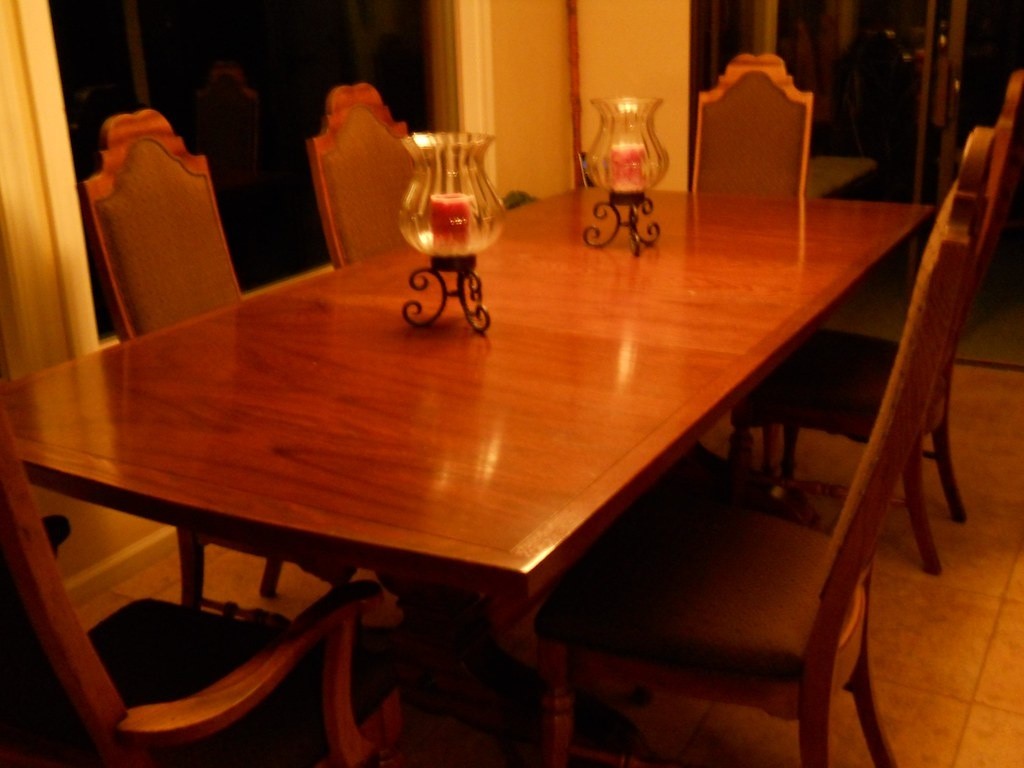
[0,54,1024,768]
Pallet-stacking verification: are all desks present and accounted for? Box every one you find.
[0,189,940,767]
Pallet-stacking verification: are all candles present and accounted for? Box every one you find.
[431,193,470,250]
[614,145,645,192]
